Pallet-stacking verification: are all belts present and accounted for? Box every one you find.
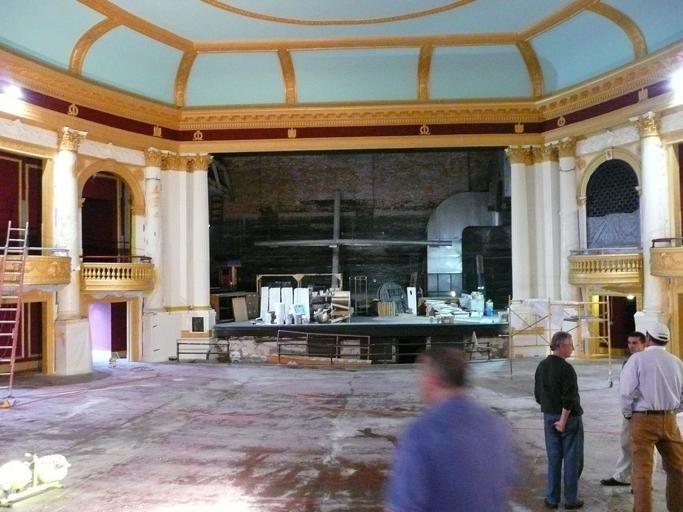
[632,409,674,414]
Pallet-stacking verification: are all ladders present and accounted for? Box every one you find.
[0,220,29,397]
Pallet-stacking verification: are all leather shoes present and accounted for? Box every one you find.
[544,497,558,509]
[565,500,584,510]
[600,477,630,486]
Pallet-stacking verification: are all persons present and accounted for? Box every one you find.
[534,332,584,509]
[385,347,521,512]
[600,322,683,512]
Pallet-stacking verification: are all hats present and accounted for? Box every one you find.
[646,323,670,342]
